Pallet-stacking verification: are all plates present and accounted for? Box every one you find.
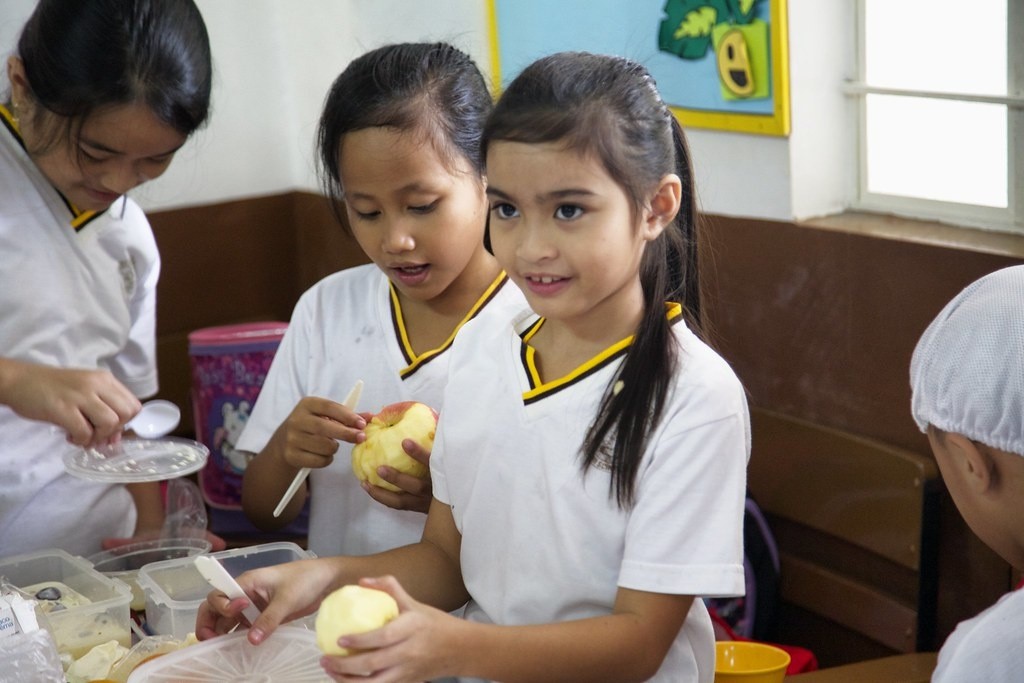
[56,437,210,485]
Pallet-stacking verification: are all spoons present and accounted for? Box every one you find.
[49,398,180,440]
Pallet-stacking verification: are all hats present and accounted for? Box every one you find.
[909,263,1024,458]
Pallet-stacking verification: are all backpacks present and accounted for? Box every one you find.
[706,491,793,646]
[187,321,312,537]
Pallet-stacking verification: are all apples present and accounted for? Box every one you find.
[351,400,441,491]
[316,584,398,657]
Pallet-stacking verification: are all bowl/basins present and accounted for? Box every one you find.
[0,539,319,666]
[714,641,790,683]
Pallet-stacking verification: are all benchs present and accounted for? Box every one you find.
[748,406,940,683]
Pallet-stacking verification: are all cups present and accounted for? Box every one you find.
[185,322,311,538]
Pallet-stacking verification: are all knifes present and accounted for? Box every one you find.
[274,381,362,518]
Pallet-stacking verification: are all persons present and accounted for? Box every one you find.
[908,263,1023,683]
[237,42,535,559]
[195,51,754,683]
[0,0,213,558]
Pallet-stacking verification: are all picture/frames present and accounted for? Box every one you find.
[483,0,792,140]
